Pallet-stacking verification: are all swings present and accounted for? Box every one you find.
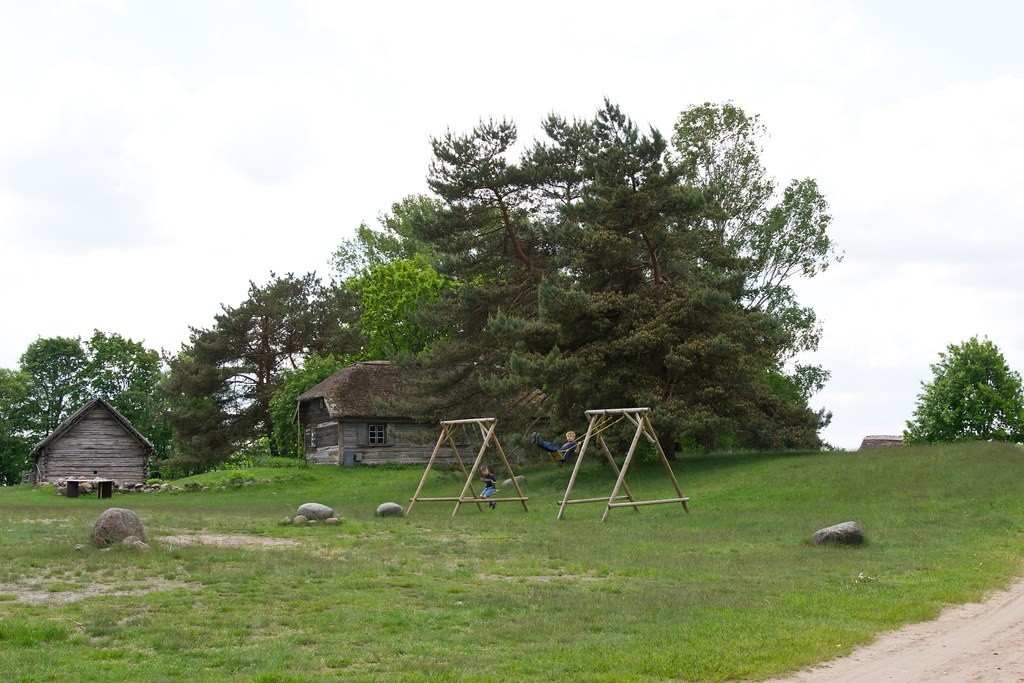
[547,417,624,463]
[463,424,500,496]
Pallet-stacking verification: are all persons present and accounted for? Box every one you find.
[532,430,582,463]
[480,465,496,510]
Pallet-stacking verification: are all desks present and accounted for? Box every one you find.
[65,479,114,499]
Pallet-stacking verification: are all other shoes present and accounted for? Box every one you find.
[492,502,496,509]
[489,502,492,507]
[531,432,537,444]
[535,433,540,445]
